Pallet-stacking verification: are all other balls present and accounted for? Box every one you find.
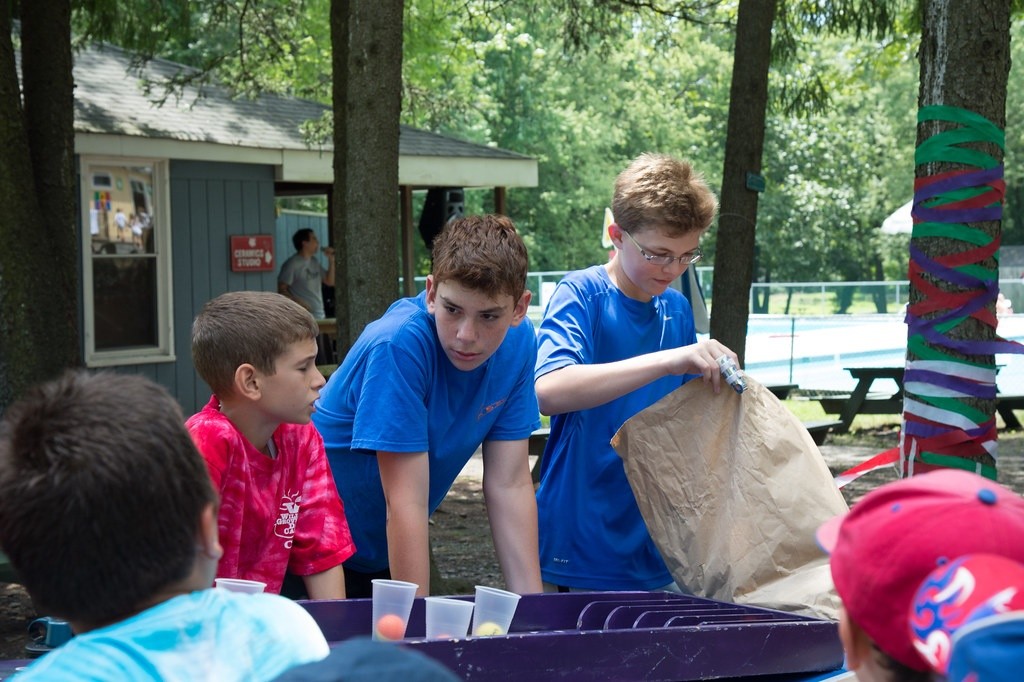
[376,614,405,641]
[475,622,503,637]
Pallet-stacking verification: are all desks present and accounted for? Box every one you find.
[832,364,1024,435]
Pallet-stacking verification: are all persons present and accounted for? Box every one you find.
[138,207,150,225]
[282,213,544,599]
[276,227,335,365]
[534,153,749,594]
[0,371,330,682]
[184,290,358,600]
[128,214,142,254]
[815,469,1023,682]
[111,208,129,242]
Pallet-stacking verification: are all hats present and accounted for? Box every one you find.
[909,553,1024,682]
[815,467,1024,668]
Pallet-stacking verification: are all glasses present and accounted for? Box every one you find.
[620,228,705,268]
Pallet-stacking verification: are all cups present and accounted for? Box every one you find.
[370,578,419,642]
[425,597,476,639]
[471,585,523,635]
[215,577,267,595]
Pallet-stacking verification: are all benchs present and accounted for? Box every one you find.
[809,393,1024,413]
